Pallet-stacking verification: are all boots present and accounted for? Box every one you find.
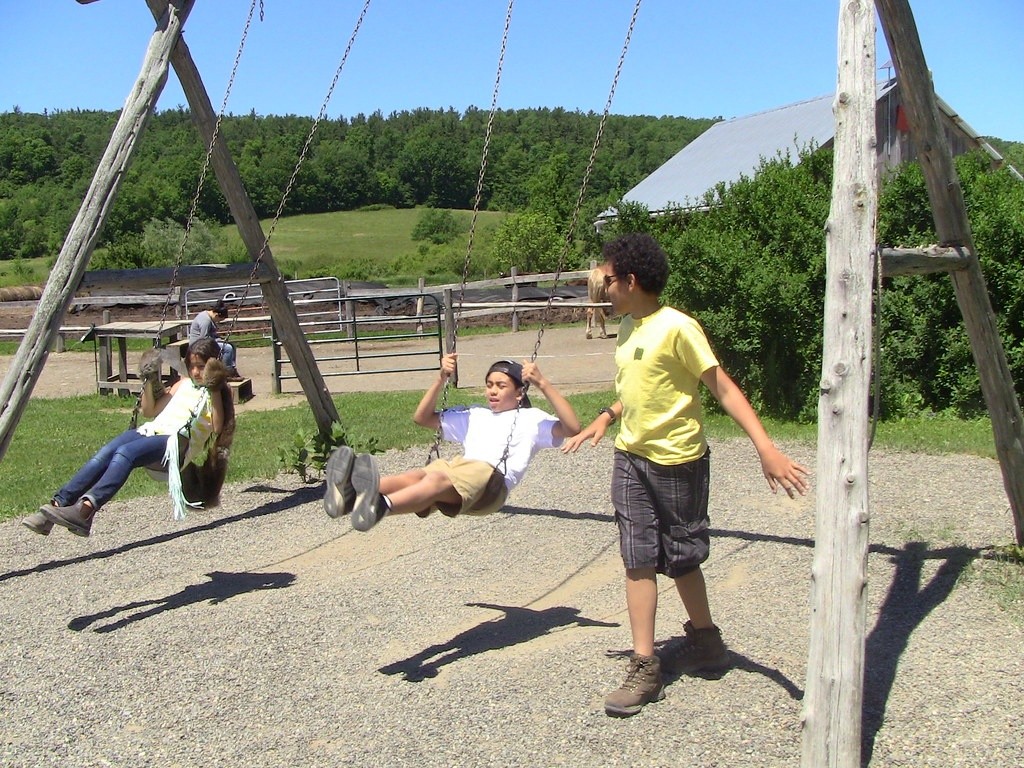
[227,367,244,382]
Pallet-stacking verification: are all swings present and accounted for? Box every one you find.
[413,0,642,515]
[125,0,373,475]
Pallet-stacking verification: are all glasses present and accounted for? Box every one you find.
[605,274,618,285]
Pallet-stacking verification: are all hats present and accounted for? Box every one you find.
[486,359,532,408]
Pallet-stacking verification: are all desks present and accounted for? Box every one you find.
[96,321,182,396]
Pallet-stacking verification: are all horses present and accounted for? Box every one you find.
[584,265,621,340]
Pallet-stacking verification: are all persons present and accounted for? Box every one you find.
[22,338,225,537]
[324,352,581,532]
[189,300,245,382]
[560,233,812,714]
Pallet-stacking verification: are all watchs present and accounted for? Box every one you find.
[599,407,616,425]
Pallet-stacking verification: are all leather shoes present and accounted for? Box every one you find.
[40,500,95,537]
[22,500,58,536]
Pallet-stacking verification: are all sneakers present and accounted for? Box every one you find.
[662,621,729,674]
[324,446,393,532]
[604,654,666,714]
